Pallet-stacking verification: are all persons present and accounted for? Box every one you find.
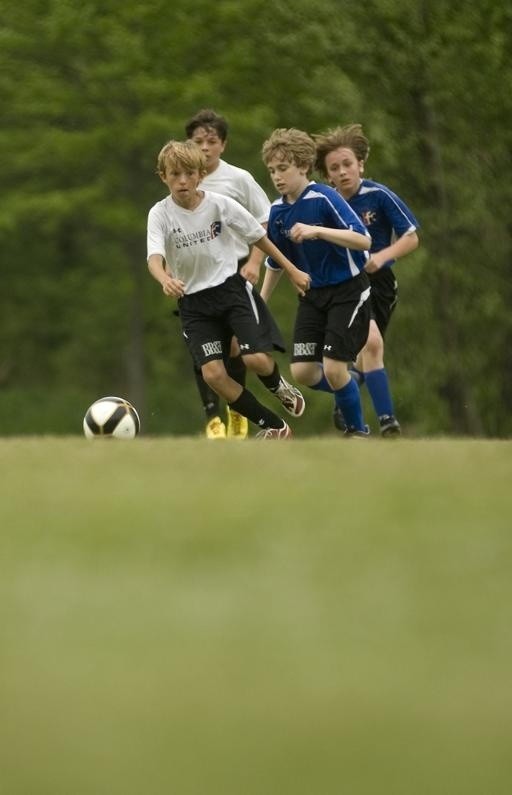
[258,128,374,437]
[311,123,420,437]
[147,139,311,440]
[186,110,271,438]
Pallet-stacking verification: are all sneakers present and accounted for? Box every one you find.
[225,403,248,439]
[270,376,306,418]
[207,416,225,439]
[334,370,363,430]
[380,415,400,437]
[341,424,371,439]
[255,420,293,441]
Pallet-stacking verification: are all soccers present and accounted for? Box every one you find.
[83,397,140,440]
